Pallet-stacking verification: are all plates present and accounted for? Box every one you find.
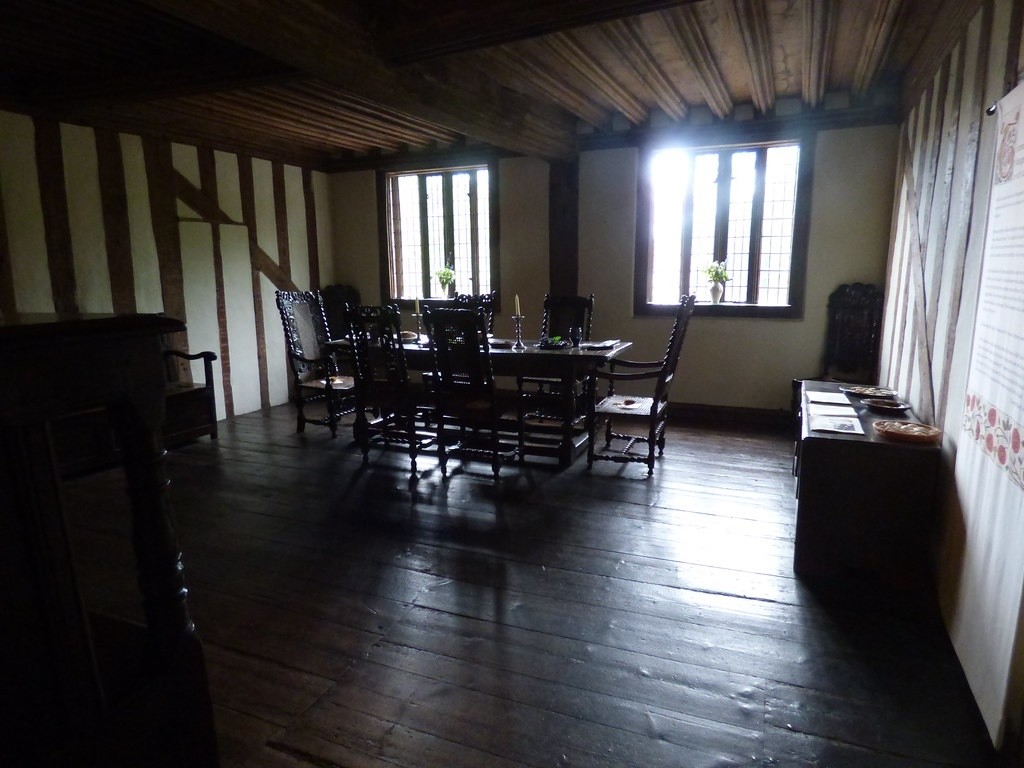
[838,385,898,398]
[860,398,911,415]
[872,420,941,443]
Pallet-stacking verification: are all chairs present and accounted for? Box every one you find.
[340,293,594,479]
[273,291,378,438]
[585,294,696,476]
[0,319,221,767]
[813,282,884,384]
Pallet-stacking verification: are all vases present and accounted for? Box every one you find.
[711,280,725,304]
[440,286,447,299]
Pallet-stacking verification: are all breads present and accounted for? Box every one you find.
[400,331,416,338]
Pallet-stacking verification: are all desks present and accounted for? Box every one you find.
[788,379,943,589]
[323,332,633,468]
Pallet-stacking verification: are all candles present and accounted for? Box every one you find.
[514,294,521,316]
[414,297,420,314]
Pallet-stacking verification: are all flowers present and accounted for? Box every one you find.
[436,267,455,289]
[709,261,727,284]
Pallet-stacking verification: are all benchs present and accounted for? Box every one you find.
[0,311,215,484]
